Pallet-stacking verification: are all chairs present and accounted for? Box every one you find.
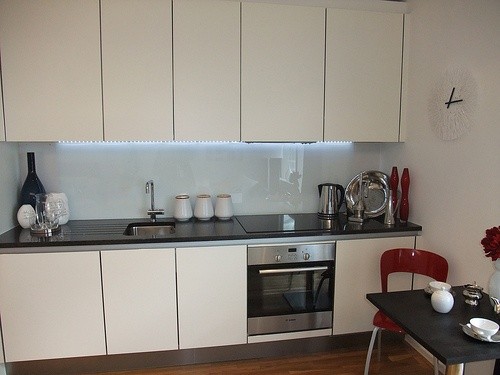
[364,247,451,375]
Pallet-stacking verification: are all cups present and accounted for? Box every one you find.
[45,192,70,225]
[35,193,59,229]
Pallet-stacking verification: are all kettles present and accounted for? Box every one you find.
[317,183,345,219]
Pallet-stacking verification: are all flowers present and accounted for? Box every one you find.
[481,225,500,261]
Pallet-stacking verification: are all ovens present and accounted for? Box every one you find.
[247,239,334,345]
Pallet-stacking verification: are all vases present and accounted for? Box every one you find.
[488,258,500,307]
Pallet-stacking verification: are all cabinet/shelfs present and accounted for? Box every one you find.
[0,234,416,375]
[0,0,412,144]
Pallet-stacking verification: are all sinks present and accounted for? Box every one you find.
[123,221,176,236]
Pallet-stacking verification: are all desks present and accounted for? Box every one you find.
[365,285,500,375]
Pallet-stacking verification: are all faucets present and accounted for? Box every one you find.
[144,179,157,222]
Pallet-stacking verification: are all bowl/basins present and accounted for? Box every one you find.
[345,171,390,218]
[429,281,452,292]
[469,318,499,339]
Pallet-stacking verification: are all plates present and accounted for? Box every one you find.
[463,323,500,343]
[424,285,457,296]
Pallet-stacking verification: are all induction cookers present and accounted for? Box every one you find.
[236,213,339,235]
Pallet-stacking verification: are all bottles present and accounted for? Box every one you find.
[431,287,454,313]
[20,151,47,224]
